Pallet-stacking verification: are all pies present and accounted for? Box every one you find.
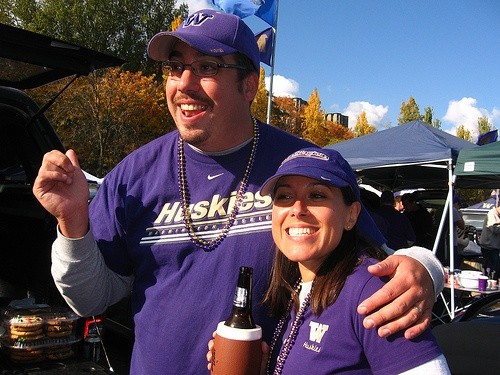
[7,316,73,341]
[9,346,74,361]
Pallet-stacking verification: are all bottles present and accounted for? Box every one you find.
[225,266,258,329]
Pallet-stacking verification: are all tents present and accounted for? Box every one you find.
[326,118,480,320]
[432,141,500,255]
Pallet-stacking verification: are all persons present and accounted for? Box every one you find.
[206,148,451,375]
[480,201,500,279]
[453,196,465,267]
[401,193,433,249]
[374,191,416,251]
[32,9,445,375]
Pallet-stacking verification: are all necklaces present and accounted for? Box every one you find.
[267,277,313,375]
[178,115,260,250]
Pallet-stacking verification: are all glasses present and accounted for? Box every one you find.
[161,58,247,79]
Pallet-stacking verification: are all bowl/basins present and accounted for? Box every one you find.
[16,304,51,313]
[458,274,489,289]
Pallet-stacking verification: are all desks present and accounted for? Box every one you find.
[431,283,500,325]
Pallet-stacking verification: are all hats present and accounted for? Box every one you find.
[260,147,359,201]
[147,8,261,73]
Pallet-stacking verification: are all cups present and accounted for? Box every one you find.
[478,276,488,291]
[487,280,498,289]
[210,320,263,375]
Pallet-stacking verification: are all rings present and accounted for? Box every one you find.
[414,306,424,316]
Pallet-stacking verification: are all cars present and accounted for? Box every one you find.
[0,22,129,251]
